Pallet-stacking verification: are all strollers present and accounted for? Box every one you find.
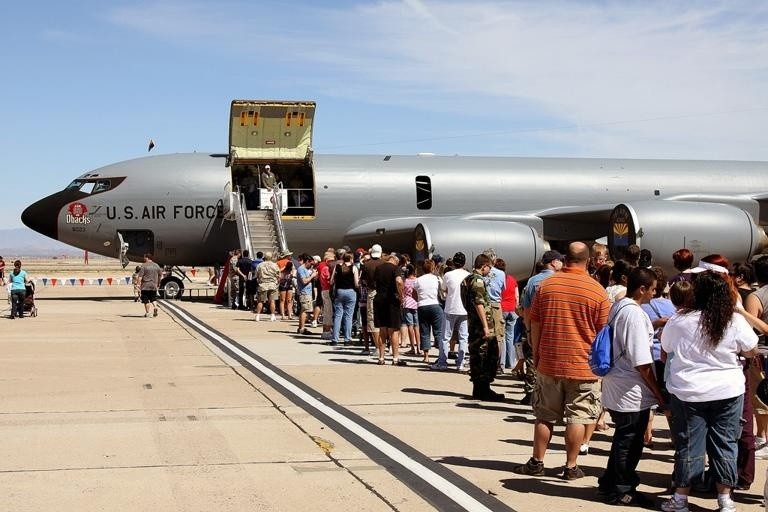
[8,281,39,318]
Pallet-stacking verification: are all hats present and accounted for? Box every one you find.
[278,249,293,258]
[542,250,566,264]
[682,261,728,274]
[370,244,383,258]
[264,164,271,169]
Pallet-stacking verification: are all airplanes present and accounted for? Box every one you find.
[19,101,768,296]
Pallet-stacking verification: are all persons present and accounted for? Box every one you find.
[242,165,308,210]
[0,255,5,286]
[6,260,28,319]
[133,266,141,301]
[139,253,163,317]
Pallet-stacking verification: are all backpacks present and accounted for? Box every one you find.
[586,303,642,379]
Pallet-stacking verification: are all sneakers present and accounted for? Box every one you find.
[660,497,691,512]
[377,359,385,365]
[326,341,338,346]
[344,341,357,346]
[430,362,449,371]
[404,349,416,355]
[715,498,738,512]
[281,314,322,334]
[358,350,370,355]
[255,314,260,321]
[621,490,654,508]
[270,314,277,322]
[754,434,768,461]
[578,444,589,455]
[144,308,158,318]
[393,359,406,365]
[10,314,24,319]
[593,484,620,506]
[561,463,587,480]
[229,300,272,313]
[513,458,547,476]
[457,364,471,373]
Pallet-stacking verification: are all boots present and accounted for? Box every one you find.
[472,383,505,402]
